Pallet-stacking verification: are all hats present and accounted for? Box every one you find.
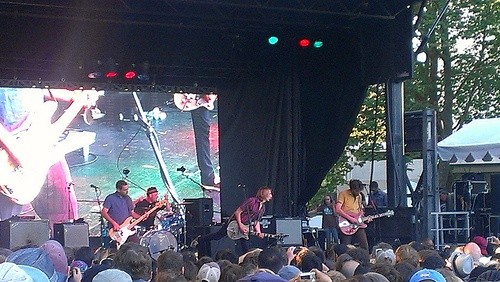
[495,247,500,254]
[334,243,347,255]
[198,262,220,282]
[92,269,132,282]
[423,256,445,269]
[147,187,158,195]
[302,255,322,271]
[473,236,488,254]
[410,268,446,282]
[0,262,50,282]
[448,252,476,279]
[286,246,297,265]
[277,266,301,281]
[6,247,58,282]
[377,249,396,267]
[39,240,68,273]
[349,179,363,190]
[494,238,500,244]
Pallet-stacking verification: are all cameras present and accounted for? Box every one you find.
[69,268,77,276]
[487,237,493,242]
[300,272,313,281]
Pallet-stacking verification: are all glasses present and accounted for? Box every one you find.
[324,199,329,200]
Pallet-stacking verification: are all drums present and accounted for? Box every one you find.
[141,230,178,264]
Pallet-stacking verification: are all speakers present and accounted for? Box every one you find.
[186,198,213,226]
[187,225,235,258]
[54,222,89,247]
[269,217,303,246]
[0,219,49,251]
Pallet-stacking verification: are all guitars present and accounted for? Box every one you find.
[227,220,289,245]
[338,209,394,235]
[108,200,167,250]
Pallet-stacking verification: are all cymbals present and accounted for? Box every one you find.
[135,207,156,217]
[91,211,101,214]
[177,201,194,205]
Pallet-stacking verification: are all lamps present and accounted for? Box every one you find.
[88,63,137,81]
[263,31,330,48]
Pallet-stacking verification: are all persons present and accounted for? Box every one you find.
[0,230,500,282]
[335,180,373,255]
[308,195,339,249]
[191,94,220,191]
[101,180,150,253]
[190,186,273,255]
[133,186,170,237]
[440,190,467,243]
[367,181,388,208]
[0,88,99,237]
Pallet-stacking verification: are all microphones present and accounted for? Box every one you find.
[91,185,98,188]
